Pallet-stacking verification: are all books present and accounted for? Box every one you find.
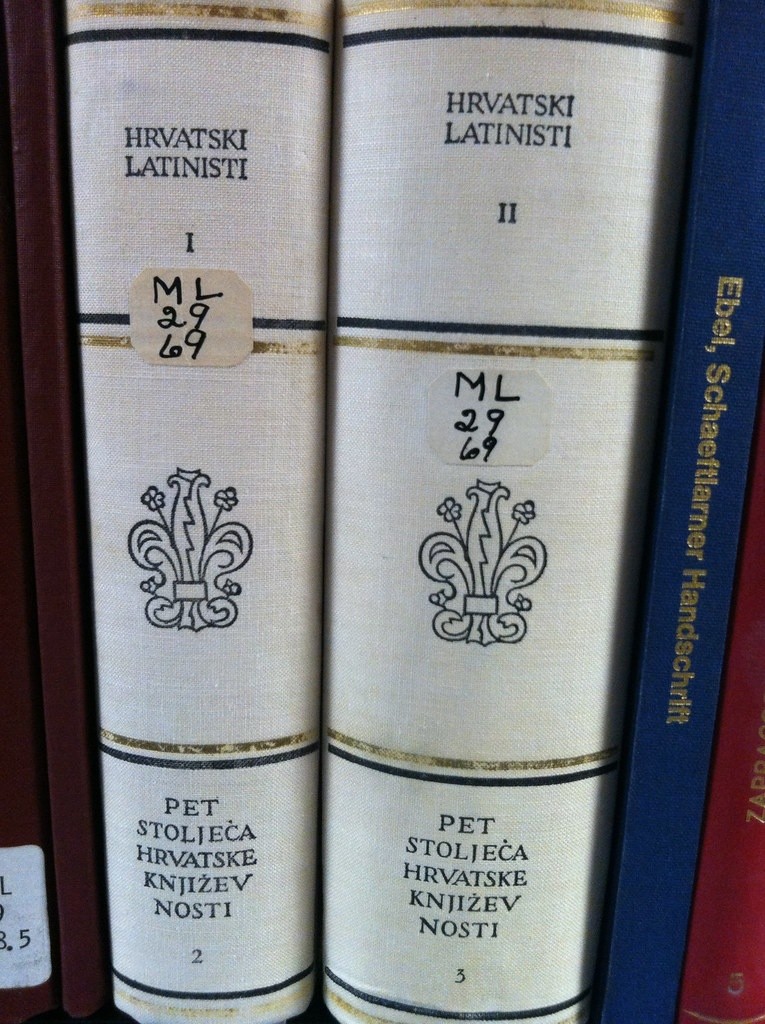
[3,0,111,1024]
[600,0,765,1024]
[320,0,700,1024]
[65,0,335,1024]
[0,348,56,1024]
[678,398,765,1024]
